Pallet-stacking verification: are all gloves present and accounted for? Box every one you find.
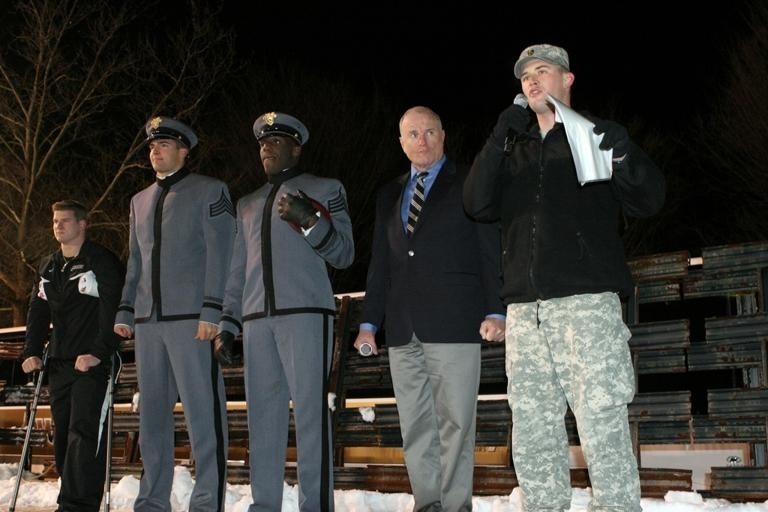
[212,330,236,368]
[277,189,318,229]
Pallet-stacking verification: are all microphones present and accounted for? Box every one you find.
[359,343,373,356]
[504,93,529,157]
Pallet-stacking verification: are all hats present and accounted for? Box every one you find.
[144,116,198,152]
[252,111,311,147]
[514,44,570,79]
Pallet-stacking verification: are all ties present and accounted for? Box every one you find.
[406,170,428,240]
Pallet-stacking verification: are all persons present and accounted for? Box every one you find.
[20,199,127,512]
[354,105,505,512]
[113,115,235,512]
[214,112,356,512]
[460,42,669,512]
